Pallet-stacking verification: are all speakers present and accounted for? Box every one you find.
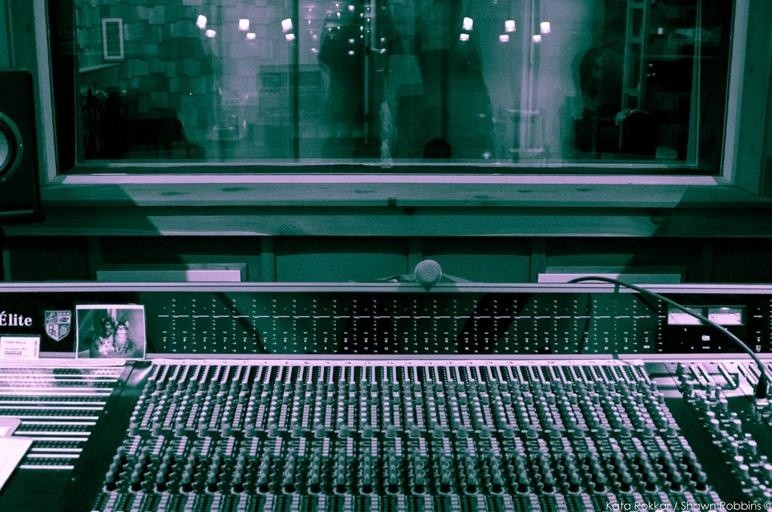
[0,67,43,225]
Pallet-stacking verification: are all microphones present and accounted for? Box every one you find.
[413,260,445,287]
